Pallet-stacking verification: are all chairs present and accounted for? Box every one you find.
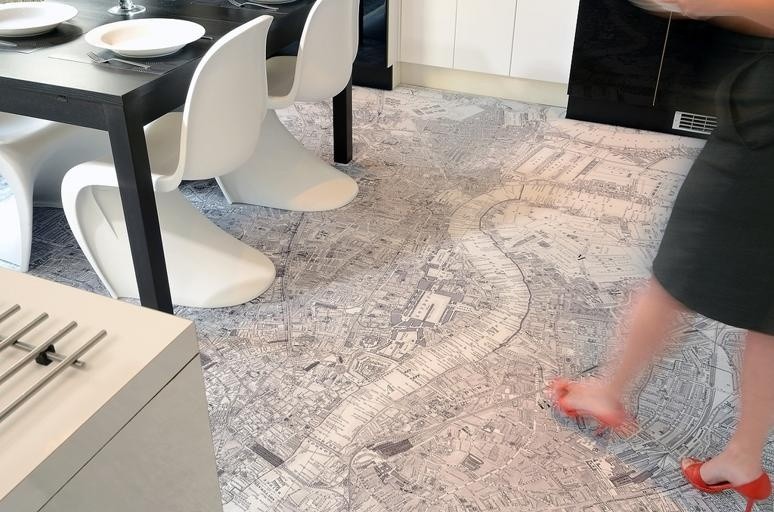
[215,0,361,215]
[57,12,279,306]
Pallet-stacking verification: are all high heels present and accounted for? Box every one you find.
[554,378,624,435]
[678,453,774,509]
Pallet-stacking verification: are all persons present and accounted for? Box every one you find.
[552,0,774,512]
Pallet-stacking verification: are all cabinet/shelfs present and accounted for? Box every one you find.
[0,267,225,512]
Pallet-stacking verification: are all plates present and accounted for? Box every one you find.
[83,17,207,60]
[0,2,80,38]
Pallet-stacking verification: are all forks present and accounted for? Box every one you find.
[227,0,280,11]
[85,50,151,69]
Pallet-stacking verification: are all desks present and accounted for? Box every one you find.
[0,0,352,315]
